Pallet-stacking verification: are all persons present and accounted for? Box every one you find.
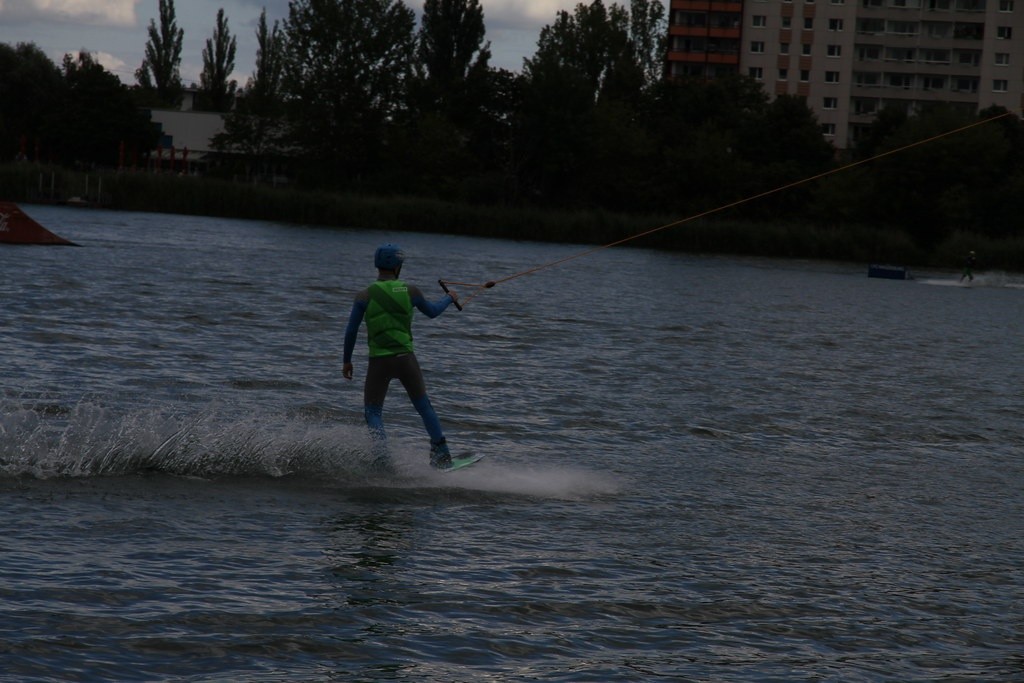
[960,251,976,282]
[342,245,459,471]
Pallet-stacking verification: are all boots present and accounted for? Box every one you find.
[429,437,453,468]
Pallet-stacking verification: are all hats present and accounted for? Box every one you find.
[375,241,413,266]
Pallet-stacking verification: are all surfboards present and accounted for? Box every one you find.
[352,450,486,478]
